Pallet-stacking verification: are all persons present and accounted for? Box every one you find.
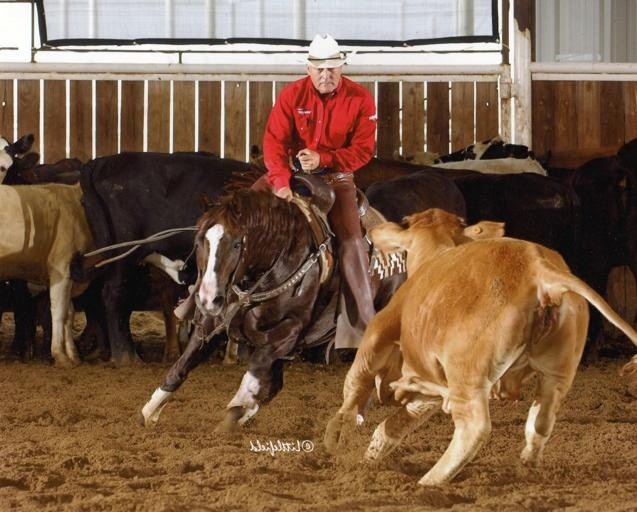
[247,33,376,350]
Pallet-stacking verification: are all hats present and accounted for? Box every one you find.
[305,32,358,70]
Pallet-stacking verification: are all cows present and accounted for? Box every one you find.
[0,133,637,370]
[322,207,637,489]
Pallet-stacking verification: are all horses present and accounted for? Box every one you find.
[140,180,407,436]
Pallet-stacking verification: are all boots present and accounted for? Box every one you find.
[341,238,378,337]
